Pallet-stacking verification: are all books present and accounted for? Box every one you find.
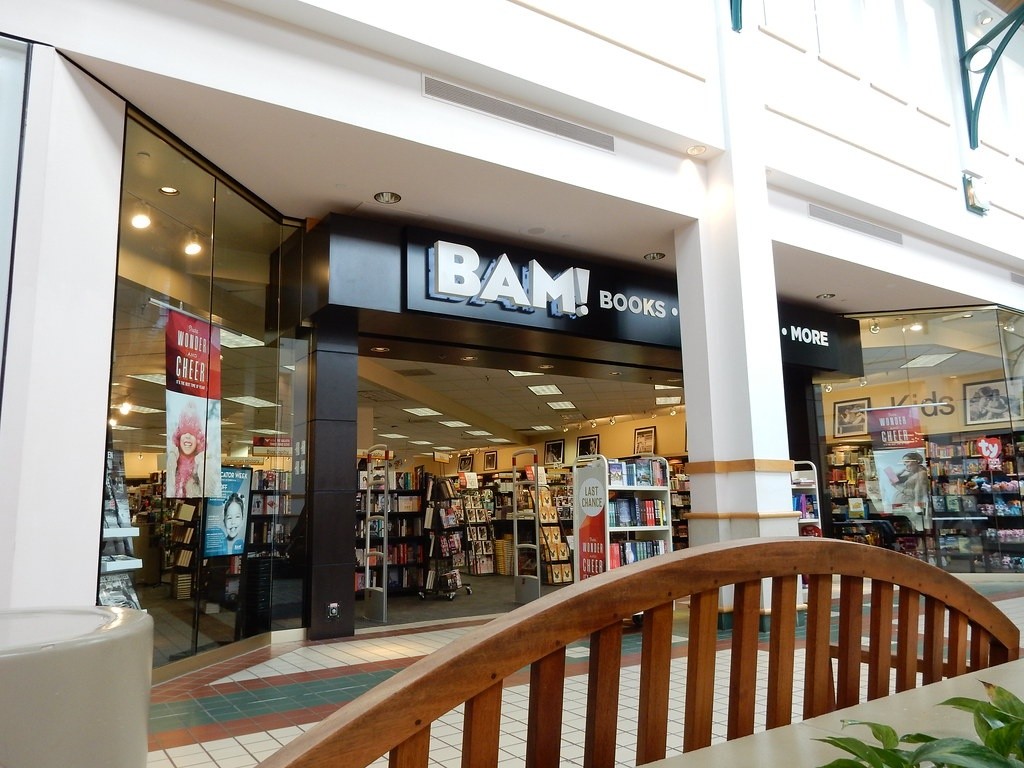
[606,459,664,487]
[247,470,293,558]
[826,448,867,499]
[355,518,424,592]
[609,490,667,526]
[610,539,668,570]
[792,492,819,519]
[356,469,421,513]
[926,440,978,512]
[171,504,196,599]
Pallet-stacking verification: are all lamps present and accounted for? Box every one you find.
[184,228,202,255]
[577,424,583,429]
[1004,319,1015,332]
[449,451,452,458]
[859,378,867,386]
[131,200,151,229]
[475,448,480,454]
[466,449,470,455]
[457,450,461,457]
[563,426,569,433]
[977,11,993,26]
[650,412,656,418]
[591,422,596,428]
[823,385,833,393]
[669,408,676,416]
[609,418,615,425]
[867,318,880,335]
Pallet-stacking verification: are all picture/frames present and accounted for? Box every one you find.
[576,433,600,461]
[544,439,565,465]
[484,451,497,471]
[962,375,1024,427]
[832,395,870,437]
[634,426,656,454]
[684,421,688,452]
[456,454,474,472]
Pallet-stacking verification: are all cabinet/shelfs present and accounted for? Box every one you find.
[97,426,1024,619]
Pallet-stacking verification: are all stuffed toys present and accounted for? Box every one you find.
[964,474,1024,571]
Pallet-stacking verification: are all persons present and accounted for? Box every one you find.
[222,493,245,554]
[638,433,645,454]
[167,398,219,497]
[891,451,932,530]
[838,403,865,425]
[971,386,1008,420]
[461,458,470,470]
[585,440,596,456]
[488,456,493,467]
